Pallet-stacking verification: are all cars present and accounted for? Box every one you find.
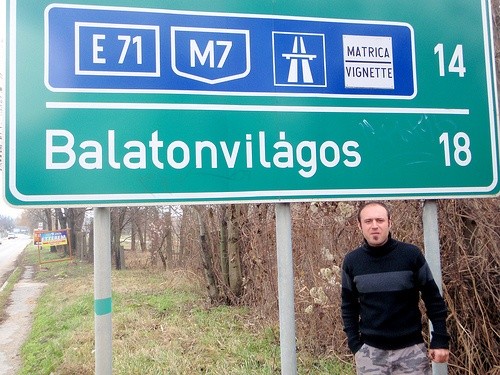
[7,232,15,239]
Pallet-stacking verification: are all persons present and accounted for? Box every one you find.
[339,201,451,375]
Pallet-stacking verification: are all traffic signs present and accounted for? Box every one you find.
[3,1,500,206]
